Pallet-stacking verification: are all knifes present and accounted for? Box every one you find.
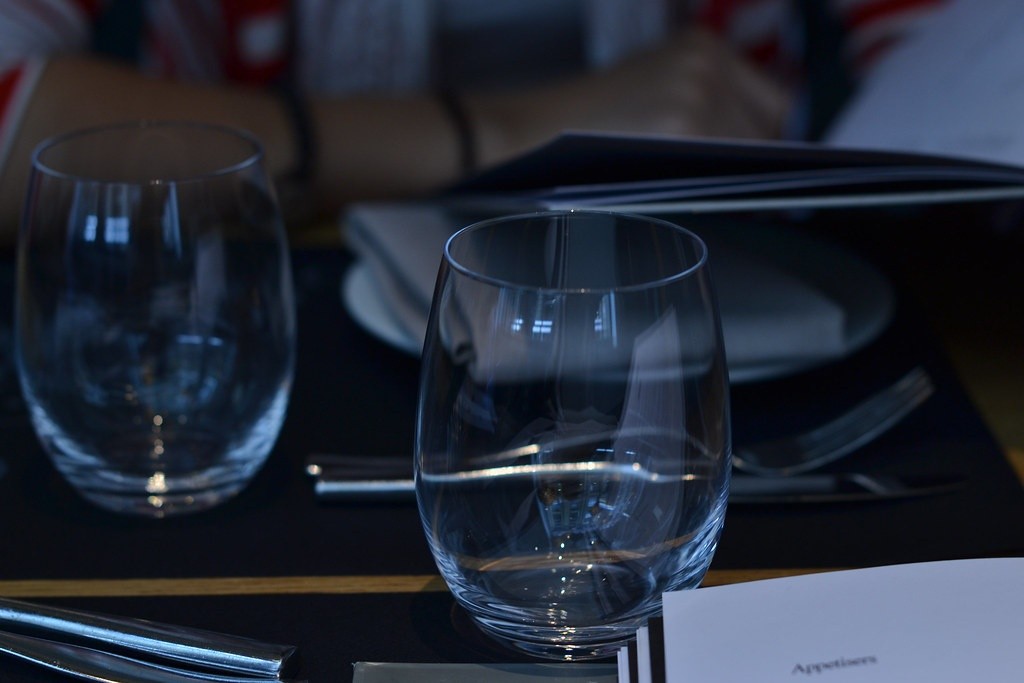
[315,471,978,503]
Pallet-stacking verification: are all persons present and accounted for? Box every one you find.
[0,1,949,238]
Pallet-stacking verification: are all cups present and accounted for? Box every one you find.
[413,211,732,662]
[14,117,297,519]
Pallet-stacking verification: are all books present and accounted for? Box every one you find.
[616,557,1024,683]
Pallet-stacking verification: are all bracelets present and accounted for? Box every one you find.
[436,84,476,178]
[276,75,316,192]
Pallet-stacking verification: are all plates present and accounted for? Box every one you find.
[341,263,889,381]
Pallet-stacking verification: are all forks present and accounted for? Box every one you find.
[303,368,935,479]
